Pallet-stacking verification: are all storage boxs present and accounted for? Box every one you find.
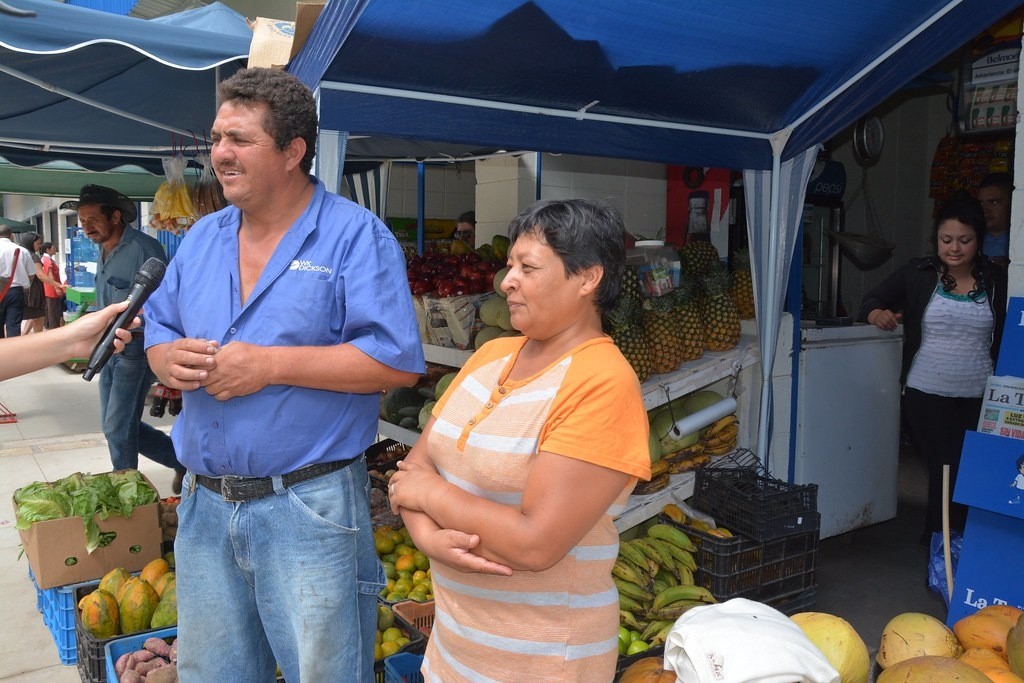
[12,436,437,683]
[611,448,822,683]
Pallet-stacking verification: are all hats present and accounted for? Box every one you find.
[60,185,137,224]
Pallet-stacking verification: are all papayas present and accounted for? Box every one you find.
[76,557,182,640]
[451,235,512,262]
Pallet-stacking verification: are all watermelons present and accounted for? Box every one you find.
[379,386,419,425]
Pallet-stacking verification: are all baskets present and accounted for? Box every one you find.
[30,445,821,683]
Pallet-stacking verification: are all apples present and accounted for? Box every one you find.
[407,251,503,298]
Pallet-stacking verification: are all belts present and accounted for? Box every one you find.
[189,452,365,503]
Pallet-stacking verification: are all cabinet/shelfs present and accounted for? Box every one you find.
[378,327,759,536]
[60,227,103,370]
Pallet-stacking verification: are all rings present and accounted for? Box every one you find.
[391,484,395,493]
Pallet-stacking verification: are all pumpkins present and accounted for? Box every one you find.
[618,657,677,683]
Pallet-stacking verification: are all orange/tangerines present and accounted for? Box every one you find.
[370,523,436,663]
[617,627,651,656]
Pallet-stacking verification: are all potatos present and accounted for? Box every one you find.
[160,503,182,538]
[114,637,180,683]
[368,444,411,532]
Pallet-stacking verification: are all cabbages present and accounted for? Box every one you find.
[14,468,158,555]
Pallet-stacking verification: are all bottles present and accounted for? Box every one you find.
[150,381,172,418]
[169,389,182,416]
[686,191,708,240]
[630,240,674,298]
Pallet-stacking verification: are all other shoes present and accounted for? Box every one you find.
[173,467,187,493]
[920,522,939,547]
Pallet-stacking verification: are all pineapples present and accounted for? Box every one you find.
[599,227,755,385]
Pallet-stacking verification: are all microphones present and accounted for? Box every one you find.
[83,257,166,382]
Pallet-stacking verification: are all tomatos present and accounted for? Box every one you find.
[165,496,182,505]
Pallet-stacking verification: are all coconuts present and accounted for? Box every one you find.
[789,605,1024,682]
[645,390,729,462]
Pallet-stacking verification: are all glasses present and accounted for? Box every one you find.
[454,230,476,241]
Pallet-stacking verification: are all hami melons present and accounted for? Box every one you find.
[418,371,460,432]
[472,268,527,350]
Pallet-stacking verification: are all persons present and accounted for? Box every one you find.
[142,67,427,683]
[454,211,475,250]
[978,171,1015,267]
[0,224,36,338]
[39,242,63,330]
[386,195,651,683]
[19,232,72,335]
[59,183,188,493]
[858,196,1006,589]
[0,300,144,382]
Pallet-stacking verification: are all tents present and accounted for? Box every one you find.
[0,216,36,233]
[1,0,536,258]
[289,0,1024,484]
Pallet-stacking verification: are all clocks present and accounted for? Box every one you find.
[853,115,884,160]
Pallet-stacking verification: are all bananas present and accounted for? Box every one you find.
[614,525,718,646]
[633,413,740,495]
